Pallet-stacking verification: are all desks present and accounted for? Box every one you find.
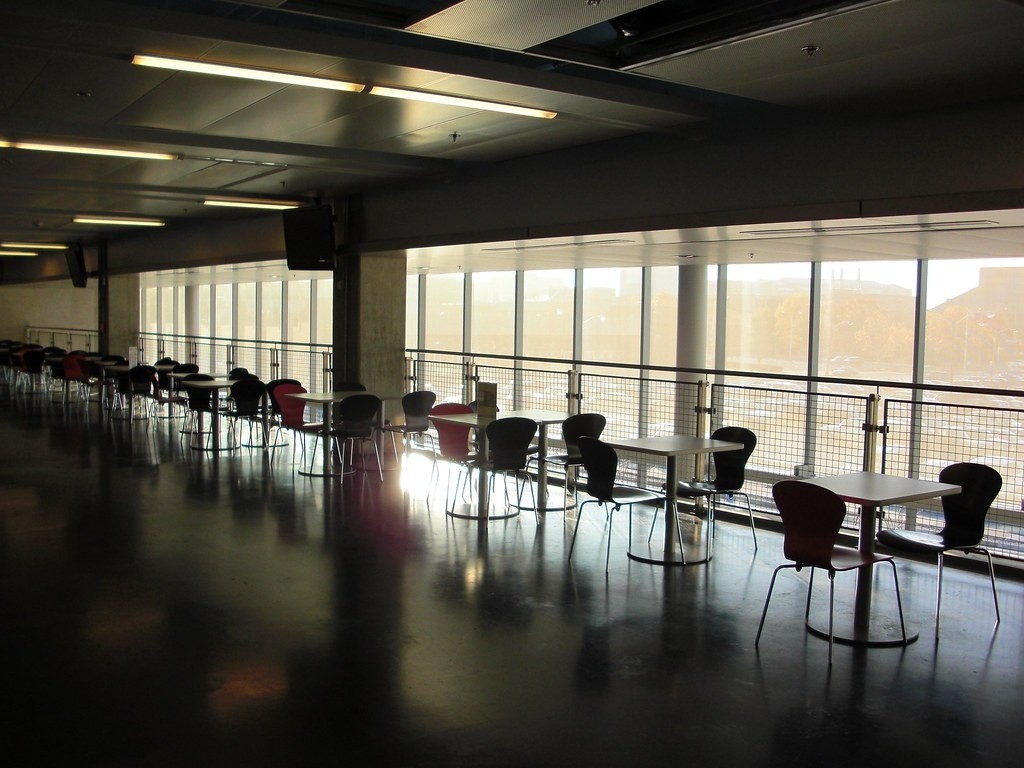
[47,357,90,404]
[105,365,150,420]
[157,365,189,418]
[499,409,577,511]
[167,373,221,433]
[242,380,290,447]
[286,391,382,477]
[349,391,406,471]
[797,471,962,646]
[89,357,126,401]
[211,373,232,422]
[607,435,745,565]
[427,409,543,517]
[180,380,241,451]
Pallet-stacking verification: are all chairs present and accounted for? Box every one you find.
[567,435,687,579]
[877,463,1003,637]
[753,480,909,665]
[647,425,759,563]
[0,341,606,526]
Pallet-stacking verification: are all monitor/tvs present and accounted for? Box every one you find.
[64,245,86,288]
[282,205,335,271]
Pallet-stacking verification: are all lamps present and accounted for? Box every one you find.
[0,242,70,257]
[0,133,184,161]
[72,214,165,226]
[202,195,299,210]
[124,54,559,121]
